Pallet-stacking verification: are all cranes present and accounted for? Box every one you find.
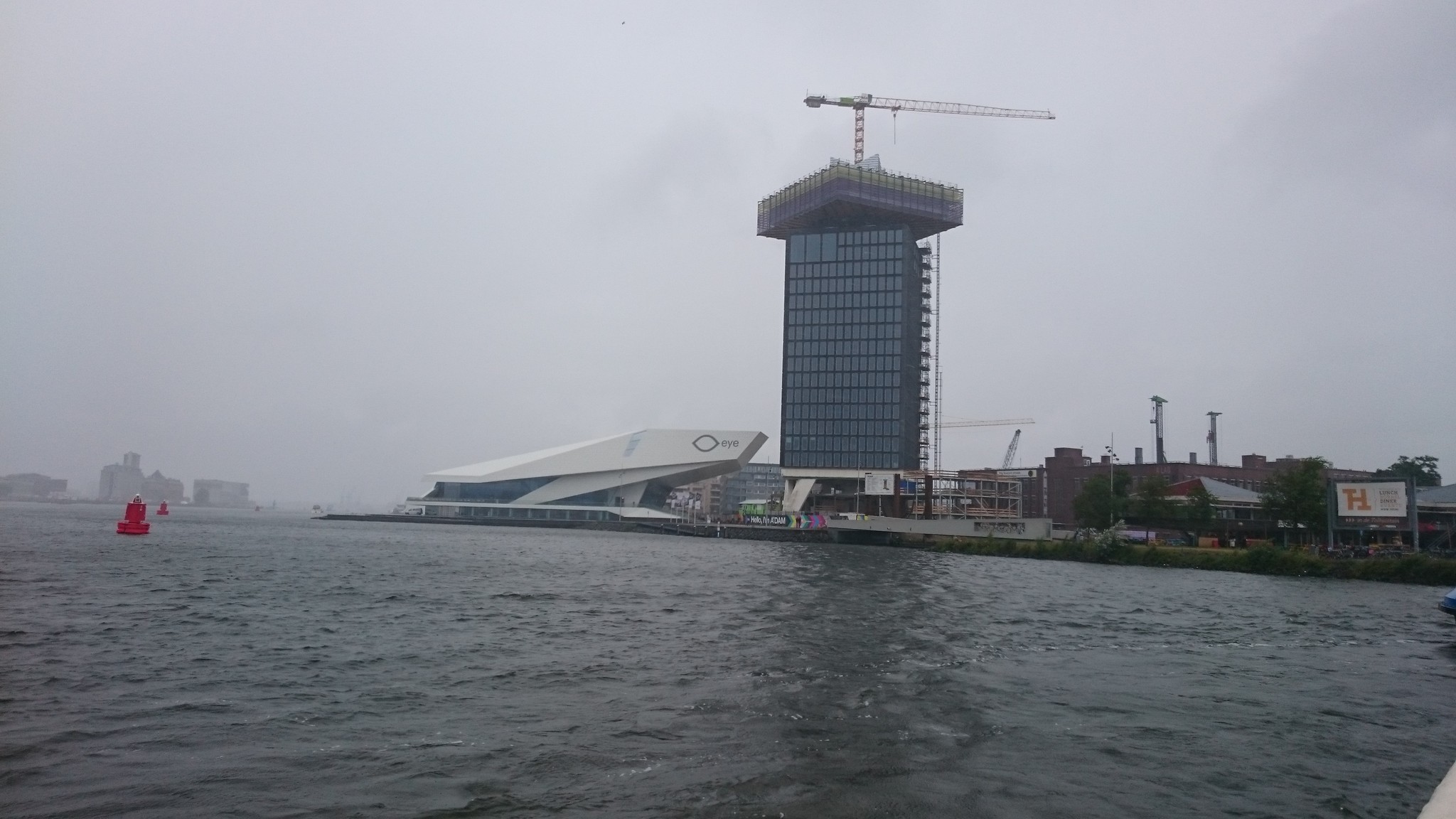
[804,93,1057,170]
[917,418,1036,492]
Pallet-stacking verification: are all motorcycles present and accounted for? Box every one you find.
[1316,543,1398,563]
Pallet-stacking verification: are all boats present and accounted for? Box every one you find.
[312,505,323,514]
[1437,585,1456,622]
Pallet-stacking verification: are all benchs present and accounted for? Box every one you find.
[1148,541,1185,544]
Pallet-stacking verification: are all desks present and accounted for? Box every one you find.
[1148,539,1183,546]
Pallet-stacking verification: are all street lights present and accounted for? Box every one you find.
[1104,432,1119,527]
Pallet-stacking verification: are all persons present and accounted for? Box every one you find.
[747,517,750,526]
[796,515,801,528]
[1228,536,1249,551]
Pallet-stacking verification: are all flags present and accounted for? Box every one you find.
[671,491,701,510]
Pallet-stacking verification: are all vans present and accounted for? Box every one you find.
[405,508,423,515]
